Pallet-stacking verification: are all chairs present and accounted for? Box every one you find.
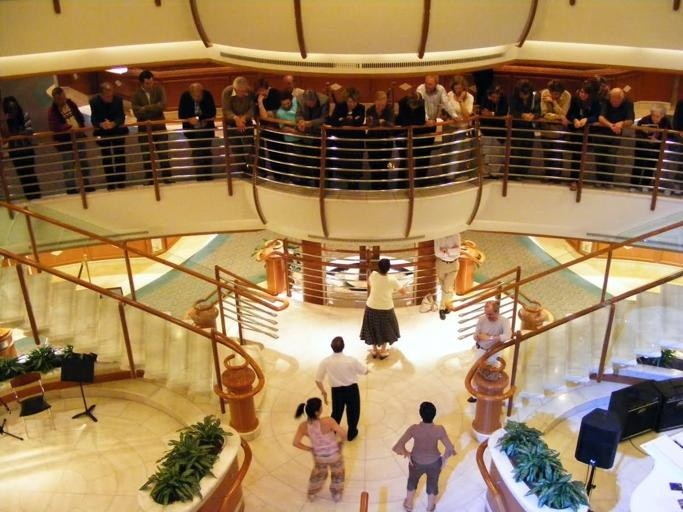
[7,371,57,440]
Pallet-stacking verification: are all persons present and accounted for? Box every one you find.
[467,302,512,403]
[0,70,683,200]
[359,259,414,360]
[314,337,372,441]
[392,402,453,511]
[292,397,346,503]
[433,234,462,319]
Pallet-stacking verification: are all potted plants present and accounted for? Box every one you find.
[135,413,243,512]
[486,416,591,512]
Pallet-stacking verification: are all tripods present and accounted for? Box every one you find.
[72,382,98,424]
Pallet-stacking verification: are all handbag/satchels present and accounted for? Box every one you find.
[419,294,434,313]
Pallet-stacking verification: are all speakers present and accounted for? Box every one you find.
[652,378,683,433]
[603,380,662,441]
[575,408,623,469]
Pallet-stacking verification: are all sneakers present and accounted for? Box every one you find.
[467,396,476,402]
[440,309,451,320]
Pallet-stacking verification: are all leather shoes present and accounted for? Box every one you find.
[371,348,391,360]
